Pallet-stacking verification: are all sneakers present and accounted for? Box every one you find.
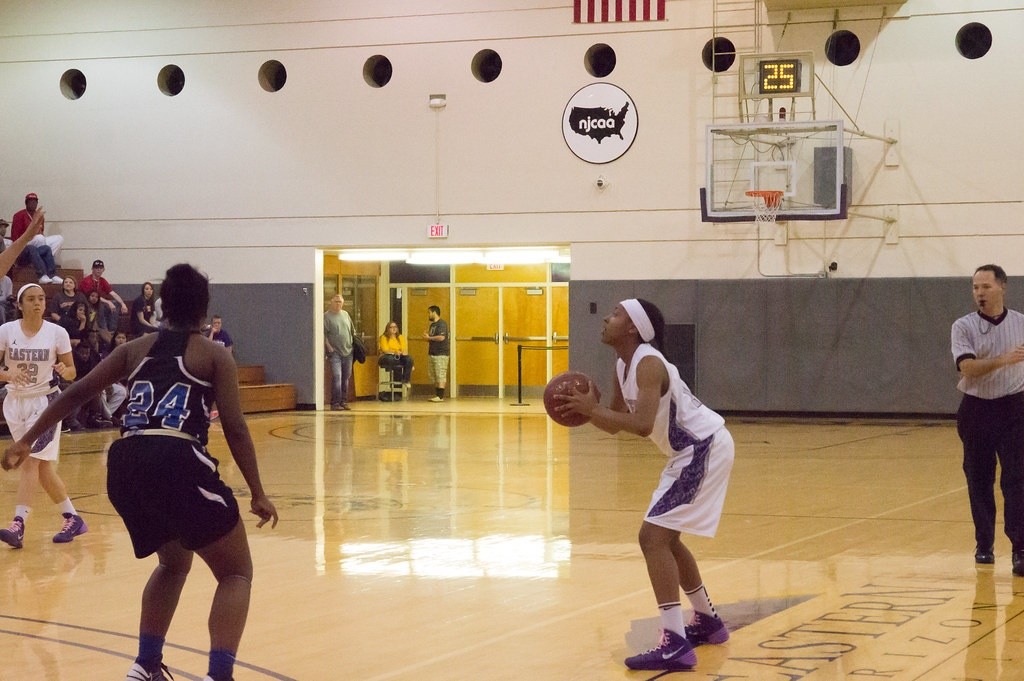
[0,516,25,548]
[427,395,443,402]
[127,654,174,681]
[684,610,729,644]
[330,402,351,411]
[624,629,697,670]
[53,513,88,543]
[39,275,63,284]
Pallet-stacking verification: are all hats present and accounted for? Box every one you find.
[26,193,38,200]
[0,219,9,226]
[93,260,104,268]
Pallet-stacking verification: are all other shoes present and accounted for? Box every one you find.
[1012,549,1024,577]
[975,545,994,563]
[87,412,113,429]
[66,418,85,431]
[107,416,123,427]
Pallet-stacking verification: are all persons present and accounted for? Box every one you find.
[0,283,88,549]
[324,295,357,411]
[378,321,412,388]
[554,298,734,670]
[0,193,233,433]
[951,264,1024,576]
[0,262,278,681]
[422,305,450,402]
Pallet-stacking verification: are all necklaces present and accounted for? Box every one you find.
[26,209,37,220]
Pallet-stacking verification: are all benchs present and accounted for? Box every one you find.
[0,261,299,433]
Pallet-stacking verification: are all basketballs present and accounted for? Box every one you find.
[543,371,603,428]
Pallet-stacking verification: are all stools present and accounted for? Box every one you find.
[376,364,410,402]
